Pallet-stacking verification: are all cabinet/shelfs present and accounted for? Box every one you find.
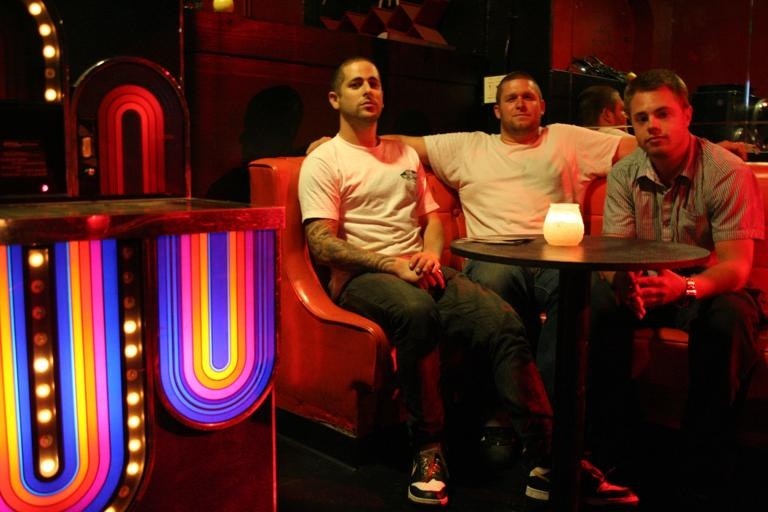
[543,202,586,246]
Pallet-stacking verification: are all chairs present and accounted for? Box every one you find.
[408,444,448,506]
[525,459,639,507]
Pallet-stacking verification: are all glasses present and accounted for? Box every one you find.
[543,202,585,247]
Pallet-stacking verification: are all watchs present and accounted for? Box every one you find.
[684,277,696,308]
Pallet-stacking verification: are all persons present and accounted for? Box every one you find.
[577,85,748,162]
[307,72,747,462]
[590,68,767,505]
[298,60,639,503]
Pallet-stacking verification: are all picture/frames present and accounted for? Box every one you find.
[0,195,287,512]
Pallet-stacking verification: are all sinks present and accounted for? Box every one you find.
[248,155,768,475]
